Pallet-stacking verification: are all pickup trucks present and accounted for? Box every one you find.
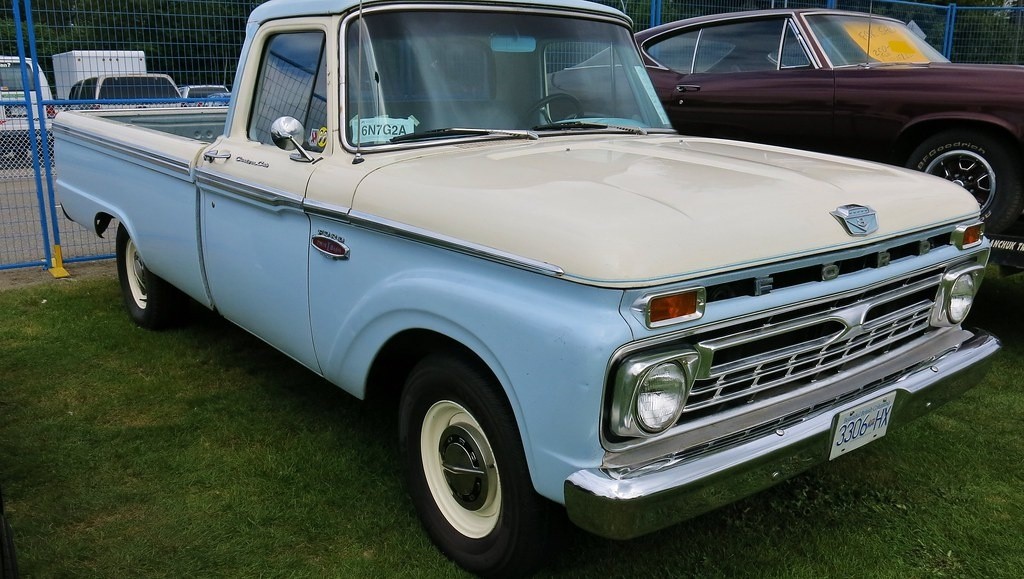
[49,0,1006,576]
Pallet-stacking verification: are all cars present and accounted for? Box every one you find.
[547,8,1024,239]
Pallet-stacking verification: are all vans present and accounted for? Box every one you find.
[68,73,181,100]
[179,84,229,98]
[0,56,58,161]
